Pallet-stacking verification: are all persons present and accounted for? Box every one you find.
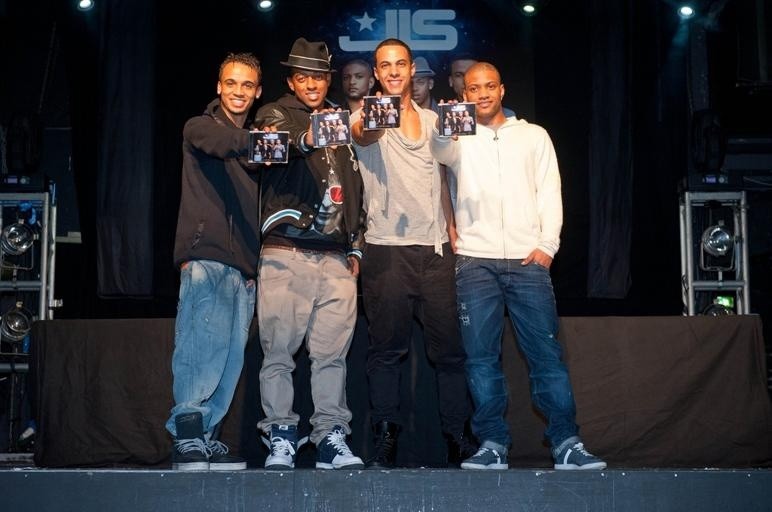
[411,56,439,112]
[347,37,478,468]
[443,110,474,135]
[317,118,349,146]
[254,138,286,162]
[164,52,277,471]
[252,36,366,470]
[341,59,376,114]
[448,59,517,117]
[367,103,398,128]
[429,60,607,471]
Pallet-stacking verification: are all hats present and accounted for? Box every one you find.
[279,38,337,73]
[412,56,436,77]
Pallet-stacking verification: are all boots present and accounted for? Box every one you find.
[448,435,480,469]
[366,422,401,470]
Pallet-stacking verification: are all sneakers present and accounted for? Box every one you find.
[264,424,298,471]
[461,448,509,471]
[209,440,248,471]
[171,412,212,472]
[315,425,365,470]
[554,442,607,471]
